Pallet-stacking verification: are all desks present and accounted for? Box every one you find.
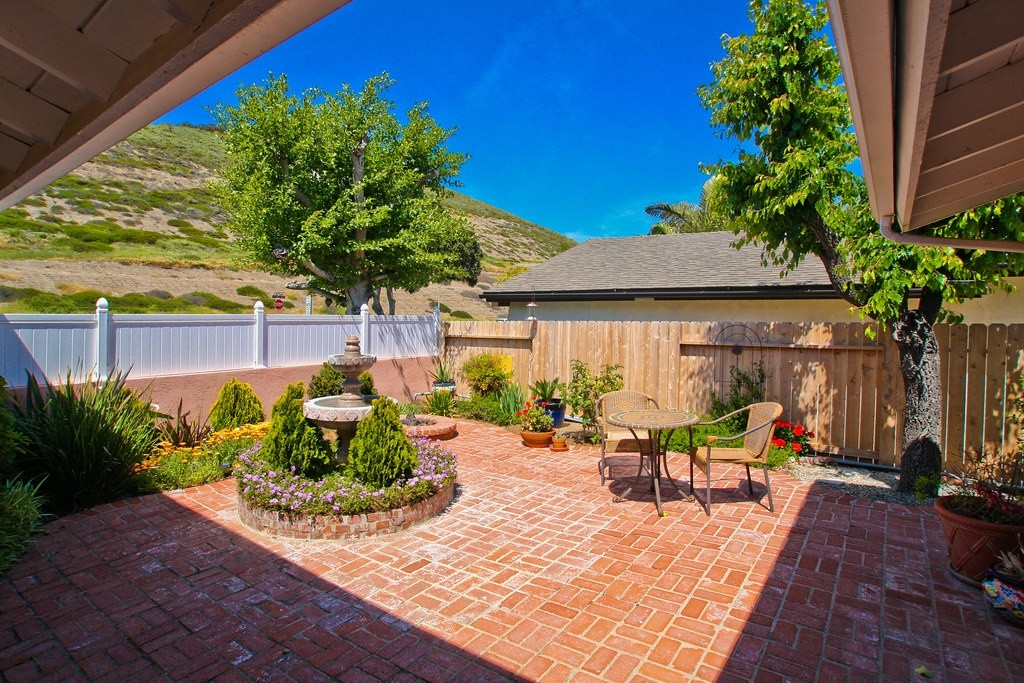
[607,409,701,517]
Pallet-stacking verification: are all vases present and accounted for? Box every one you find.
[988,562,1024,590]
[520,427,557,443]
[423,352,457,400]
[934,495,1024,581]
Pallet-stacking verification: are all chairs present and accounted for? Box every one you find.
[688,402,783,516]
[595,392,660,486]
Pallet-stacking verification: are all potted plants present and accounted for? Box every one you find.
[526,376,567,427]
[551,434,567,447]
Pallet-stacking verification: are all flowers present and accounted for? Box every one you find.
[516,396,554,432]
[939,444,1024,525]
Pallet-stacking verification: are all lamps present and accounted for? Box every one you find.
[525,286,537,321]
